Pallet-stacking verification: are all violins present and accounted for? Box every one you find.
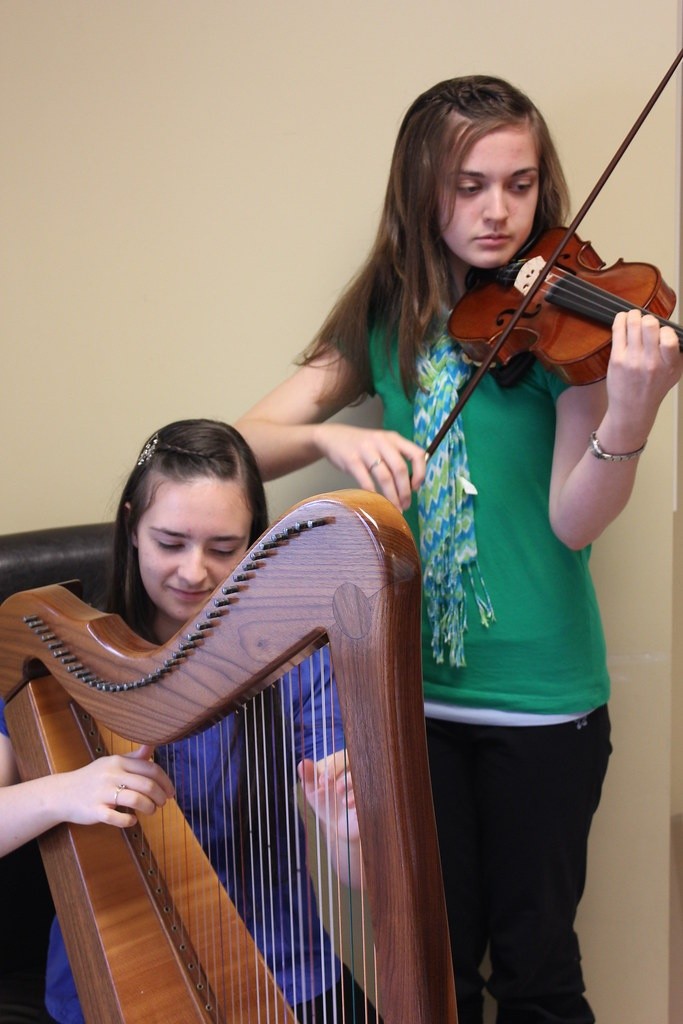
[444,223,682,392]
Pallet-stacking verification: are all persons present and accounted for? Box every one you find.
[1,418,387,1024]
[228,74,683,1024]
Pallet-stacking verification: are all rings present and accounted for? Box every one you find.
[114,784,128,806]
[369,457,384,472]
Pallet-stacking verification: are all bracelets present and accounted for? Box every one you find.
[589,431,648,462]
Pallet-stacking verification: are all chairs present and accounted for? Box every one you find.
[0,520,390,1023]
